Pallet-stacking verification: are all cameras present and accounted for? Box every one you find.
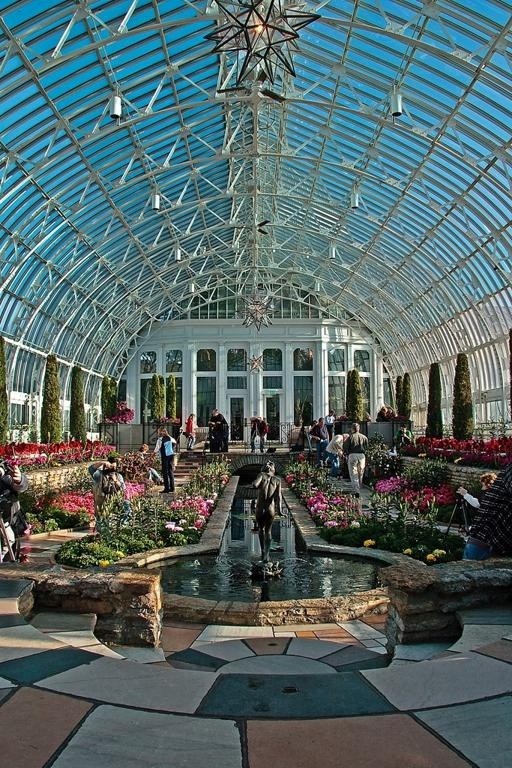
[0,460,14,476]
[102,465,115,475]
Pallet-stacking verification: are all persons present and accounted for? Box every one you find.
[0,457,28,562]
[309,409,350,477]
[185,414,196,450]
[463,464,512,561]
[208,409,229,452]
[249,417,267,453]
[343,423,368,498]
[456,472,497,509]
[136,427,178,493]
[251,460,288,563]
[394,424,412,451]
[88,456,130,531]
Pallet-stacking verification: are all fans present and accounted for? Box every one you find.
[212,64,296,109]
[237,217,271,240]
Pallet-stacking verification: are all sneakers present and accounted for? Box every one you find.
[354,493,359,498]
[187,449,194,451]
[158,487,175,493]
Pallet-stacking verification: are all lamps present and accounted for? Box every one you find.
[187,273,196,296]
[313,275,321,293]
[234,268,279,331]
[150,180,162,213]
[351,171,359,210]
[189,0,324,84]
[108,82,124,120]
[172,234,183,265]
[245,353,266,374]
[328,234,338,261]
